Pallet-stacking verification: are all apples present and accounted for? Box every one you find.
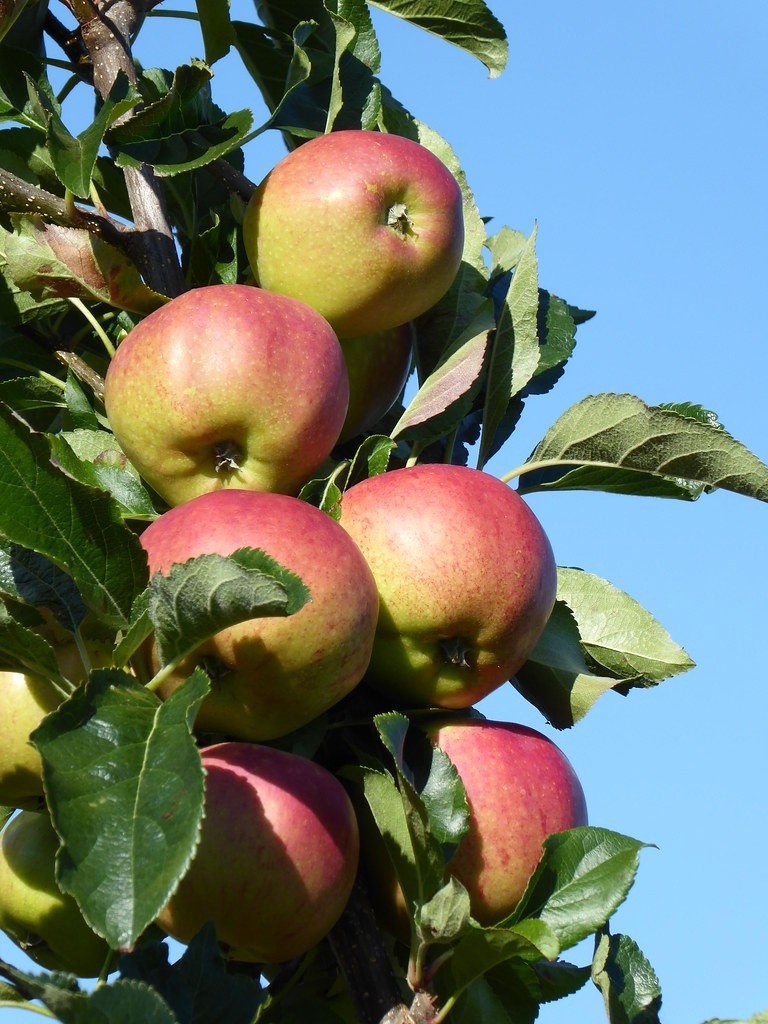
[105,283,349,509]
[329,464,557,709]
[0,602,154,979]
[241,129,465,337]
[136,489,378,741]
[335,323,413,447]
[368,717,588,924]
[150,742,358,963]
